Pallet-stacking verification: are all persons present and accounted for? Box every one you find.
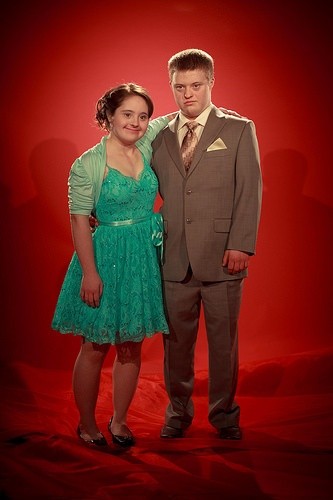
[89,49,263,440]
[52,83,250,449]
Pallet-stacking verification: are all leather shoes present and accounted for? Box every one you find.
[76,422,109,450]
[159,424,183,437]
[220,424,241,439]
[108,416,135,447]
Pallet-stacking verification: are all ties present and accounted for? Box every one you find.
[180,122,199,173]
